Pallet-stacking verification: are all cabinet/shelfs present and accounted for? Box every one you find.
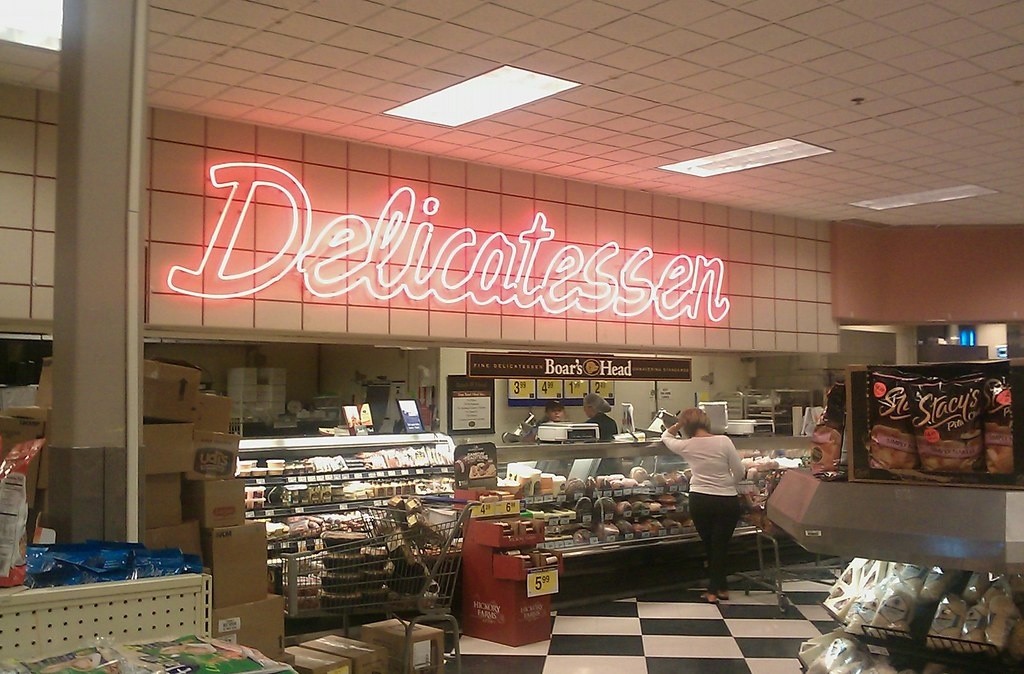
[237,432,460,556]
[495,434,813,556]
[766,358,1024,674]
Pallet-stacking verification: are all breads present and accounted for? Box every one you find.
[826,557,1024,674]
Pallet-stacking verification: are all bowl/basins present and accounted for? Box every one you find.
[245,487,266,510]
[238,460,284,477]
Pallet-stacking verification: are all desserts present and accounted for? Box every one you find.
[873,426,916,467]
[811,427,841,470]
[920,430,980,471]
[986,423,1014,473]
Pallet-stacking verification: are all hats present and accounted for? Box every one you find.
[545,400,564,412]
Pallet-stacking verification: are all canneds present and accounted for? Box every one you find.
[332,495,344,502]
[309,485,320,504]
[320,483,331,503]
[332,487,343,494]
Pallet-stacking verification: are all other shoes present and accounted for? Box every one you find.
[700,593,719,603]
[716,590,729,600]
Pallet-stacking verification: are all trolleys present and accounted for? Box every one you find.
[745,471,848,620]
[267,496,482,674]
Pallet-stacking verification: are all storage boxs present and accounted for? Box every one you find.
[0,355,445,674]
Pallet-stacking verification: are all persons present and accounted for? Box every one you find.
[582,393,618,440]
[662,408,746,602]
[541,400,569,423]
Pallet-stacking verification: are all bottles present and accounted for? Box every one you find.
[368,478,414,497]
